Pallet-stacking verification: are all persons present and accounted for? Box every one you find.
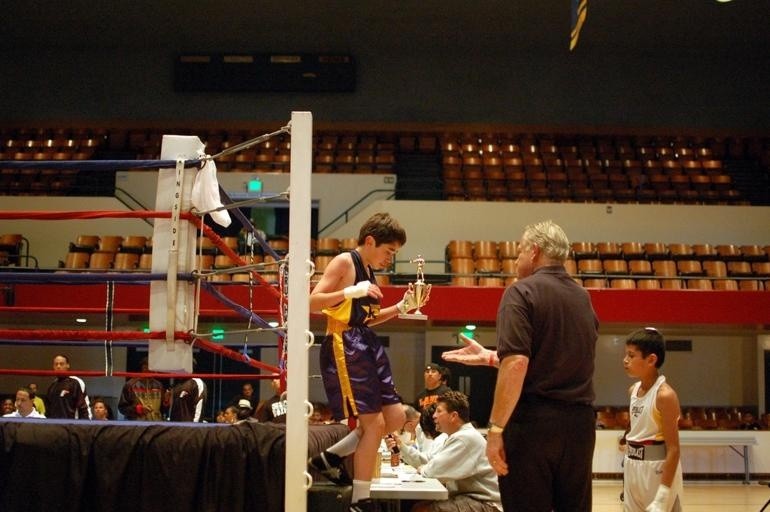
[441,218,599,512]
[408,255,424,282]
[237,218,266,256]
[307,212,433,512]
[624,329,685,512]
[1,356,339,424]
[384,363,505,512]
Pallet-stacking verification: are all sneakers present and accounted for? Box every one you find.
[307,449,351,486]
[349,497,376,511]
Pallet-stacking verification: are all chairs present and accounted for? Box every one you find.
[0,116,769,202]
[445,239,769,293]
[57,233,393,287]
[594,407,769,431]
[1,234,39,269]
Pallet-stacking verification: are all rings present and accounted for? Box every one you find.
[492,460,497,466]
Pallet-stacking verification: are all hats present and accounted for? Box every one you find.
[425,363,440,371]
[239,399,252,411]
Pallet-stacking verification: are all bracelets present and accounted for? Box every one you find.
[489,350,494,366]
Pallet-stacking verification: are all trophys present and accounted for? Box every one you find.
[398,254,433,320]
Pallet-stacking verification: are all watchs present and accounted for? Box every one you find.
[487,421,504,433]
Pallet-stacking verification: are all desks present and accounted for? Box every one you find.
[678,435,758,484]
[369,451,447,512]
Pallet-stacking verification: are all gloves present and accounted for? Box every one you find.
[646,484,672,512]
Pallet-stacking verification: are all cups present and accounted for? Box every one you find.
[391,452,399,467]
